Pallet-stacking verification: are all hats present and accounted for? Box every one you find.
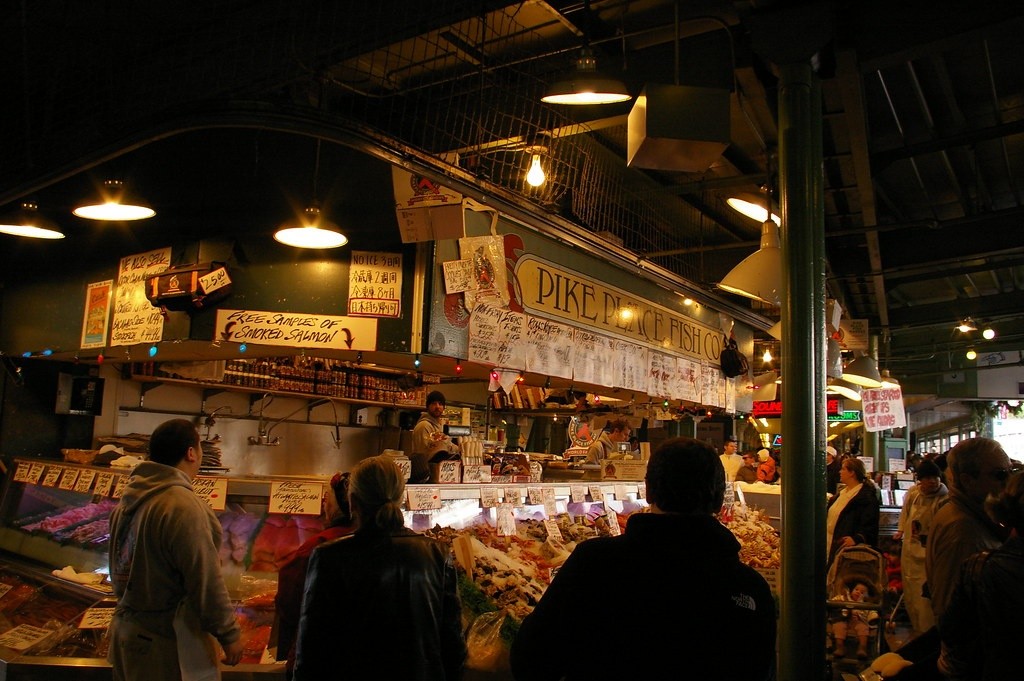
[426,391,445,405]
[826,446,837,457]
[916,459,941,479]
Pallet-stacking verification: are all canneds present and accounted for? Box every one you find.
[224,359,277,389]
[278,365,427,405]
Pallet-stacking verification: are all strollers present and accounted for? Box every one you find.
[874,535,905,633]
[824,541,889,681]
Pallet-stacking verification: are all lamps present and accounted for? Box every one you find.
[545,377,551,389]
[540,47,633,105]
[966,348,977,360]
[273,139,349,250]
[69,178,157,222]
[715,152,784,308]
[958,317,978,332]
[726,171,783,227]
[838,350,882,387]
[595,392,745,421]
[0,201,66,240]
[982,324,995,340]
[567,385,574,398]
[828,378,861,402]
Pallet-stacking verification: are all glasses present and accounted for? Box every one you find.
[980,468,1018,481]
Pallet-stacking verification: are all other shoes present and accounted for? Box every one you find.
[856,649,867,658]
[834,649,845,656]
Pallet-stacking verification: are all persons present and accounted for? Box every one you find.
[734,451,757,483]
[411,391,459,453]
[831,583,880,659]
[718,438,744,481]
[825,458,879,565]
[629,437,639,452]
[827,445,840,494]
[508,437,777,681]
[892,437,1024,681]
[754,449,775,482]
[586,421,630,462]
[105,419,243,681]
[270,456,466,681]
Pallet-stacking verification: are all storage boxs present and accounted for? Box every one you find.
[895,471,914,480]
[428,459,462,484]
[491,475,513,484]
[513,475,531,483]
[463,465,491,484]
[599,459,647,482]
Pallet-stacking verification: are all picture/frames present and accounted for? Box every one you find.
[896,479,917,490]
